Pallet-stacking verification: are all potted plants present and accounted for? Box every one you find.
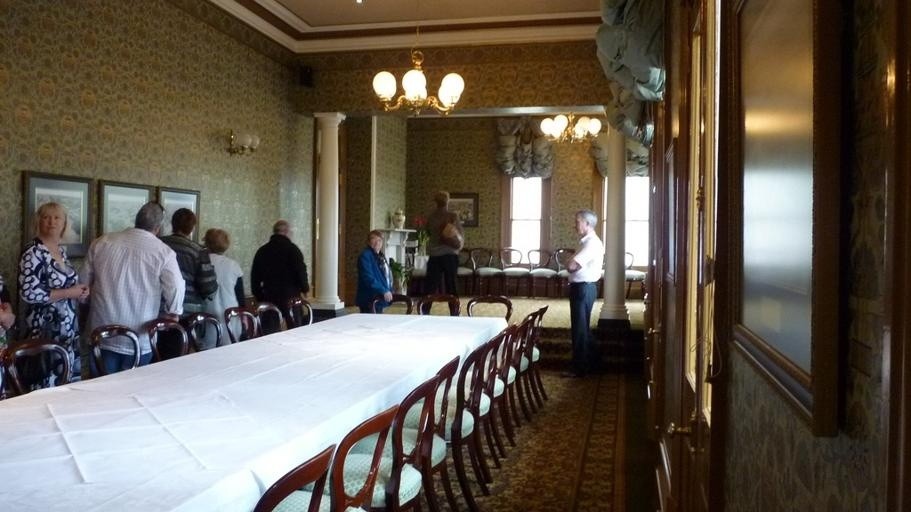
[390,258,408,297]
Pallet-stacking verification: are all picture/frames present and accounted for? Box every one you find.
[97,179,155,239]
[447,193,481,226]
[156,187,201,245]
[726,0,854,435]
[21,169,96,258]
[661,137,688,290]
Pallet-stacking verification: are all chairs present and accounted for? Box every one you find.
[224,306,258,342]
[499,246,531,298]
[372,293,413,315]
[90,324,141,373]
[512,309,542,413]
[522,303,550,400]
[285,298,313,327]
[456,247,474,297]
[253,445,335,511]
[435,331,505,468]
[185,311,224,351]
[459,323,520,448]
[1,340,73,395]
[305,374,442,511]
[495,312,534,422]
[625,252,647,298]
[417,292,461,315]
[470,248,504,296]
[468,295,512,320]
[402,341,488,511]
[255,301,283,333]
[352,354,460,511]
[150,321,188,360]
[274,402,399,512]
[528,250,558,297]
[556,248,575,295]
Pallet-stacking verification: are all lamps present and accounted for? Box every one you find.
[539,113,602,146]
[224,129,260,157]
[370,26,465,119]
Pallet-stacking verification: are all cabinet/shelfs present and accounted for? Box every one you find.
[376,228,417,293]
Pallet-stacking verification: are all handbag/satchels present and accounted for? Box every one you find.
[17,353,49,384]
[442,223,464,248]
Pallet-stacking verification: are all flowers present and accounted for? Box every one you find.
[414,215,429,243]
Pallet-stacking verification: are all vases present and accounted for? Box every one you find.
[417,244,427,256]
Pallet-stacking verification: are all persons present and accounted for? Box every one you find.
[562,209,605,377]
[250,220,309,335]
[0,274,16,402]
[78,200,186,377]
[422,190,465,316]
[355,229,394,313]
[17,202,90,391]
[157,208,218,361]
[194,228,246,350]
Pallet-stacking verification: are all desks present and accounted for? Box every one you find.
[1,314,509,512]
